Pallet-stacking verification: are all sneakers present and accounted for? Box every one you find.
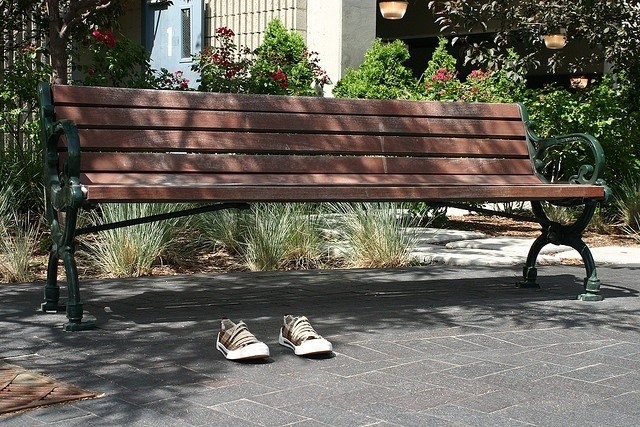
[216,319,272,362]
[279,315,334,358]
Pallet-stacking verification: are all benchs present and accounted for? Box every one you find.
[36,82,606,333]
